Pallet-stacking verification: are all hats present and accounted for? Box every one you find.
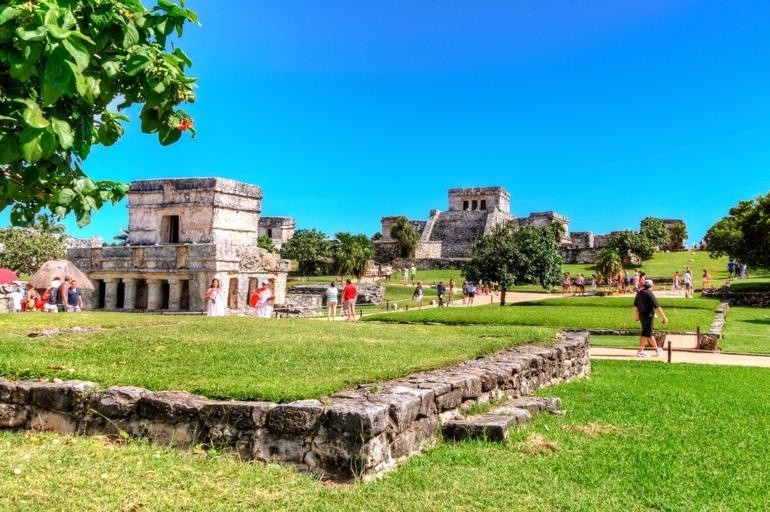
[644,279,653,285]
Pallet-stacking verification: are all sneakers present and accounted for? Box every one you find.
[637,352,646,357]
[656,348,662,356]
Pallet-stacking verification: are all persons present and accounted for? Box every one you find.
[377,263,499,310]
[7,275,83,311]
[342,279,357,323]
[253,279,275,318]
[634,279,668,357]
[325,282,338,320]
[563,259,748,296]
[205,279,227,316]
